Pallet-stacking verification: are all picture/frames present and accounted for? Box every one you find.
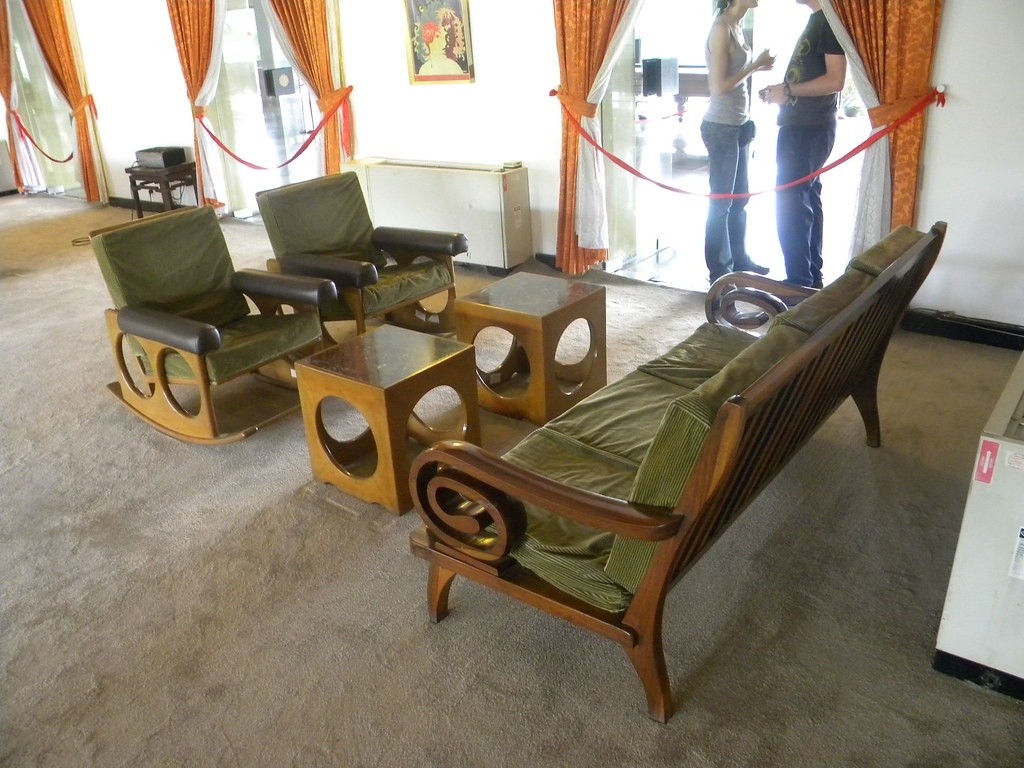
[402,0,476,86]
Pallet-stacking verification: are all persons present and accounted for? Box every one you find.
[701,0,775,281]
[759,1,846,289]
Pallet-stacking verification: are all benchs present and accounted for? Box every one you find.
[409,221,949,724]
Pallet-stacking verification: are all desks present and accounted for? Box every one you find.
[125,160,198,219]
[454,271,607,427]
[295,323,483,516]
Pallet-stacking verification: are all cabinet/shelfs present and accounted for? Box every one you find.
[934,350,1024,701]
[339,155,534,278]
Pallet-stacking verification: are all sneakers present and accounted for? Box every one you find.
[774,280,823,306]
[734,261,769,275]
[710,275,736,295]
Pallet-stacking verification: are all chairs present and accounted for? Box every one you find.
[88,206,339,446]
[255,171,469,359]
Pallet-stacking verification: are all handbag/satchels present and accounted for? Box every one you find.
[739,120,755,147]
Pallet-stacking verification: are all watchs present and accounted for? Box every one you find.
[785,83,792,97]
